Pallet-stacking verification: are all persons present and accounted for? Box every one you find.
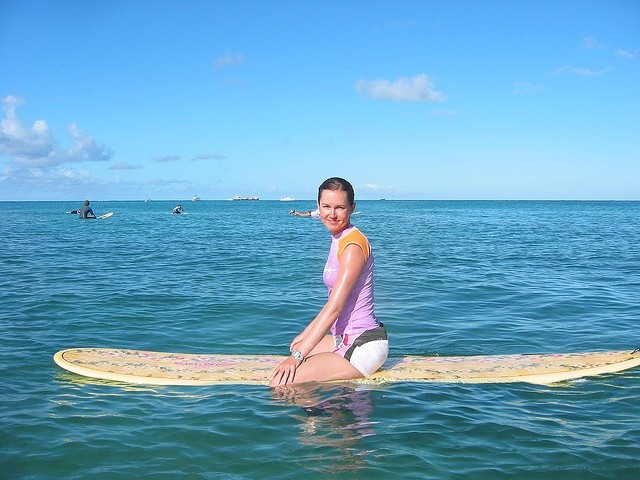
[269,176,389,388]
[79,200,97,219]
[289,208,319,218]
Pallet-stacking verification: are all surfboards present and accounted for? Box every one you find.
[96,210,113,221]
[53,347,639,386]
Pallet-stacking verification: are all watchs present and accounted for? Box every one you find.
[291,349,306,362]
